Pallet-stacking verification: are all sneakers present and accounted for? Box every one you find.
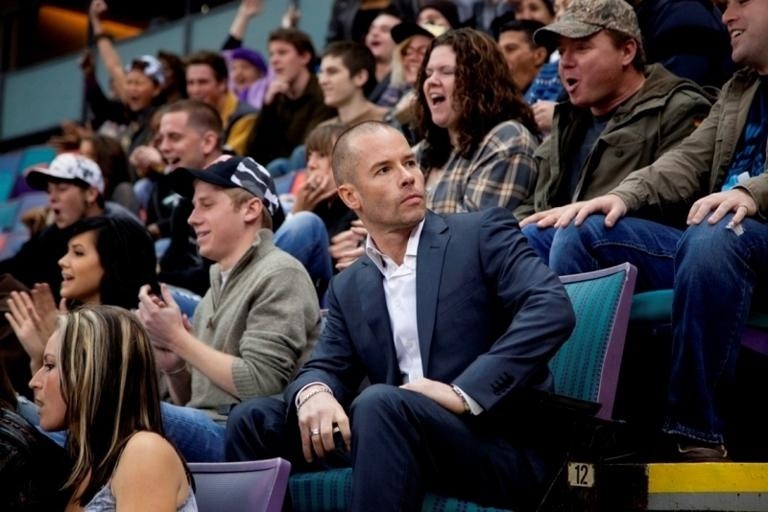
[673,436,727,463]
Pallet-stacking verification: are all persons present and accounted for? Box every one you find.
[1,1,768,512]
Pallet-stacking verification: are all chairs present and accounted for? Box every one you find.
[182,457,291,512]
[291,260,640,511]
[629,288,676,327]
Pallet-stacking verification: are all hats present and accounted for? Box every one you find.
[25,154,105,193]
[175,156,280,217]
[125,51,166,89]
[392,21,443,44]
[533,0,642,40]
